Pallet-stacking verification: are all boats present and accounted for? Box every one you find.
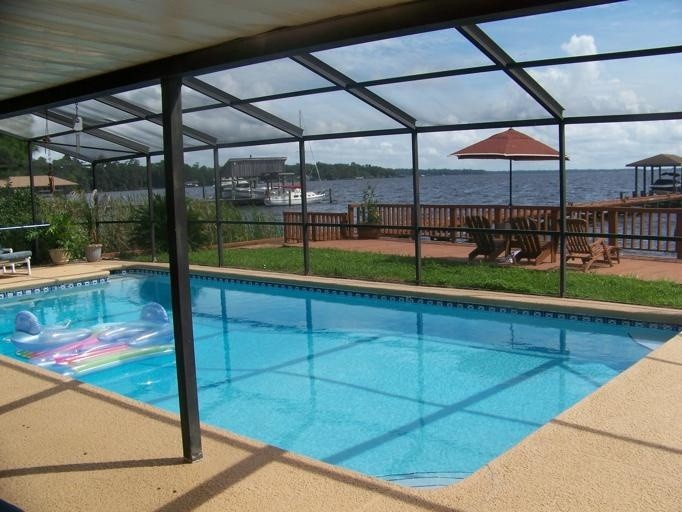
[232,186,278,200]
[648,172,681,195]
[214,177,249,191]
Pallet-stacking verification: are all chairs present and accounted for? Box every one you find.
[464,214,506,262]
[558,217,623,272]
[508,216,551,265]
[0,247,33,275]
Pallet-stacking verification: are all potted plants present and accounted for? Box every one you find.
[356,184,382,240]
[24,189,103,265]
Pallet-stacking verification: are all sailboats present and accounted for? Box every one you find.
[263,111,326,206]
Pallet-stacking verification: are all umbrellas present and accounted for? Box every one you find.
[446,128,570,219]
